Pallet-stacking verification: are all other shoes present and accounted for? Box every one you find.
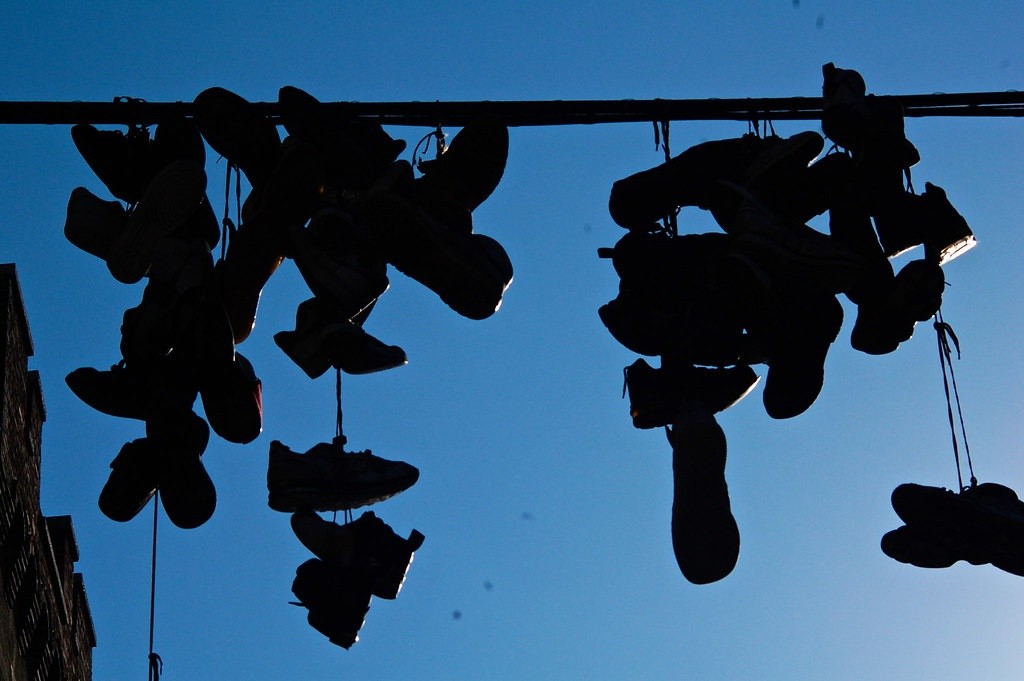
[64,65,1024,654]
